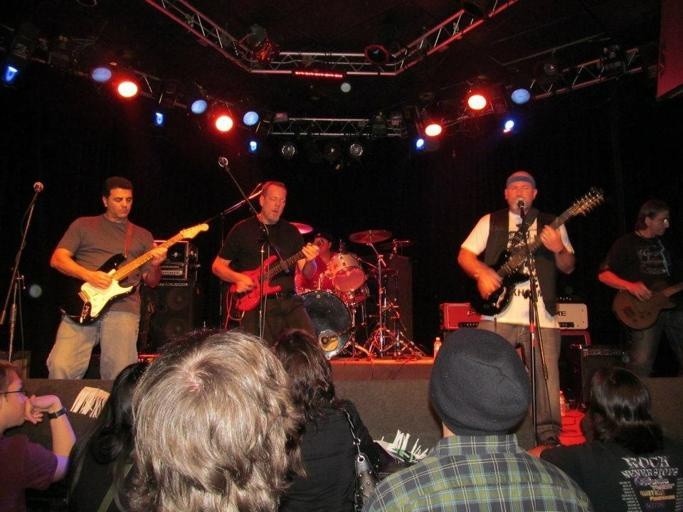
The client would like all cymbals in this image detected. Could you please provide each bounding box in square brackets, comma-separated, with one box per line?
[289, 222, 312, 234]
[379, 239, 409, 248]
[350, 230, 390, 243]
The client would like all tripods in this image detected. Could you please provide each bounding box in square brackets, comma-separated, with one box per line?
[357, 260, 416, 357]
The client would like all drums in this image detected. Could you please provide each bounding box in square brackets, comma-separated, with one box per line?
[329, 254, 365, 292]
[371, 253, 396, 275]
[295, 290, 352, 359]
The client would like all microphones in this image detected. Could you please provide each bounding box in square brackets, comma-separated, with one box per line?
[218, 156, 229, 167]
[517, 200, 525, 218]
[33, 182, 44, 192]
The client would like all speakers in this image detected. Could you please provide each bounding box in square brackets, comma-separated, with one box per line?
[158, 264, 207, 356]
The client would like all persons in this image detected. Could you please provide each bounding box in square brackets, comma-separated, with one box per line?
[46, 174, 168, 380]
[211, 181, 320, 349]
[1, 360, 76, 512]
[539, 367, 682, 512]
[361, 327, 594, 512]
[595, 197, 683, 377]
[313, 231, 338, 272]
[62, 327, 374, 512]
[457, 170, 577, 447]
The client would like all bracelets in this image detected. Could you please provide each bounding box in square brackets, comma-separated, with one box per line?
[555, 246, 567, 255]
[48, 408, 67, 418]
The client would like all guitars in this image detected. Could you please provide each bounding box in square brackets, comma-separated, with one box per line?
[62, 224, 209, 325]
[471, 188, 605, 315]
[613, 282, 682, 329]
[230, 239, 321, 310]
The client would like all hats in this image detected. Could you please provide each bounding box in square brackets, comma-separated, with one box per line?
[428, 327, 530, 437]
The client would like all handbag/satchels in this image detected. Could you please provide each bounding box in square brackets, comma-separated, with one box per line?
[353, 452, 380, 512]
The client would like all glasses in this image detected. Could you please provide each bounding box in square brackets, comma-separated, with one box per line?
[0, 387, 26, 395]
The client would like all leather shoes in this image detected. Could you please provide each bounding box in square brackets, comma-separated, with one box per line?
[535, 437, 560, 447]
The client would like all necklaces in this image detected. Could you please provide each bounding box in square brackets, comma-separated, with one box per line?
[636, 229, 644, 237]
